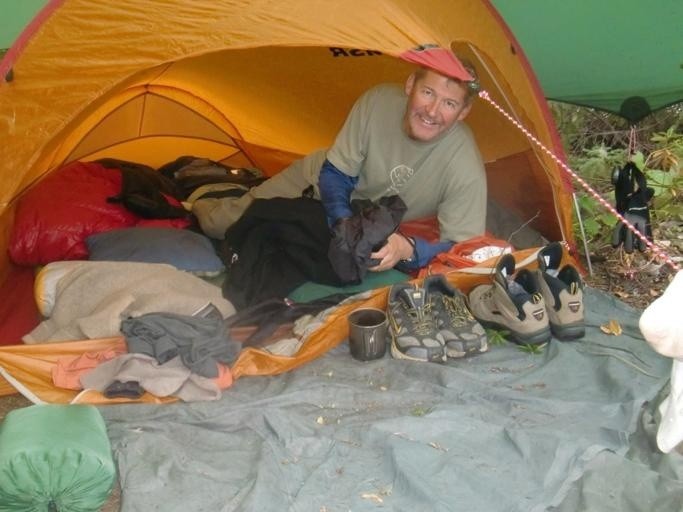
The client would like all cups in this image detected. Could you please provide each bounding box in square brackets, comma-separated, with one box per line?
[347, 307, 388, 361]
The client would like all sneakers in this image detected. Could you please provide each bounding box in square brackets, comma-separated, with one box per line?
[467, 252, 552, 346]
[530, 241, 587, 339]
[385, 281, 449, 363]
[421, 273, 488, 359]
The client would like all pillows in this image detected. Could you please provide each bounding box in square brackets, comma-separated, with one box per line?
[83, 225, 227, 278]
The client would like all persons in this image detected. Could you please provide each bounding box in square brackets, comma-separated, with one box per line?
[187, 58, 488, 273]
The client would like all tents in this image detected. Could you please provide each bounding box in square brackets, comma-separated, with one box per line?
[0, 0, 585, 405]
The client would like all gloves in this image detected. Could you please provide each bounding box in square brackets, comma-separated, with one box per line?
[610, 190, 654, 255]
